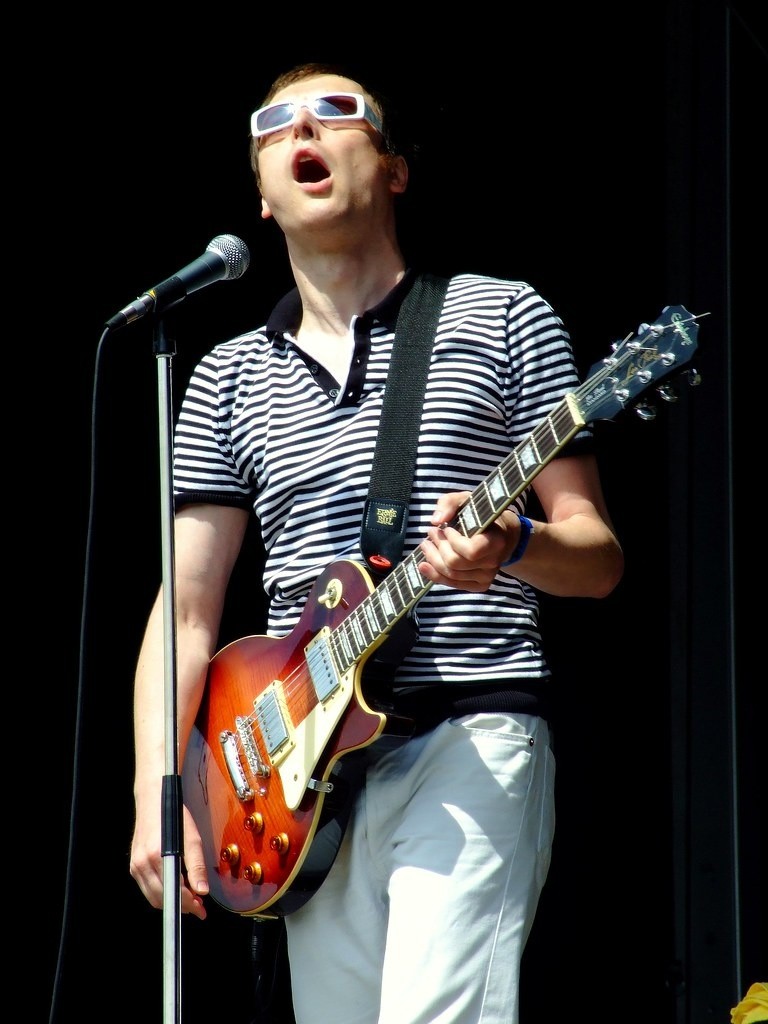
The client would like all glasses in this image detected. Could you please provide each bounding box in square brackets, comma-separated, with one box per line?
[251, 93, 395, 155]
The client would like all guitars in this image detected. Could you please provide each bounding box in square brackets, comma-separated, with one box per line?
[179, 292, 700, 926]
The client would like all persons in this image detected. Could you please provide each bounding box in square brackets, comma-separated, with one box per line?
[129, 64, 626, 1024]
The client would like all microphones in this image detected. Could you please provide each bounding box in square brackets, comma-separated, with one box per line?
[103, 234, 250, 332]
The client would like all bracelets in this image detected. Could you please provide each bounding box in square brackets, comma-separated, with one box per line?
[503, 514, 535, 569]
